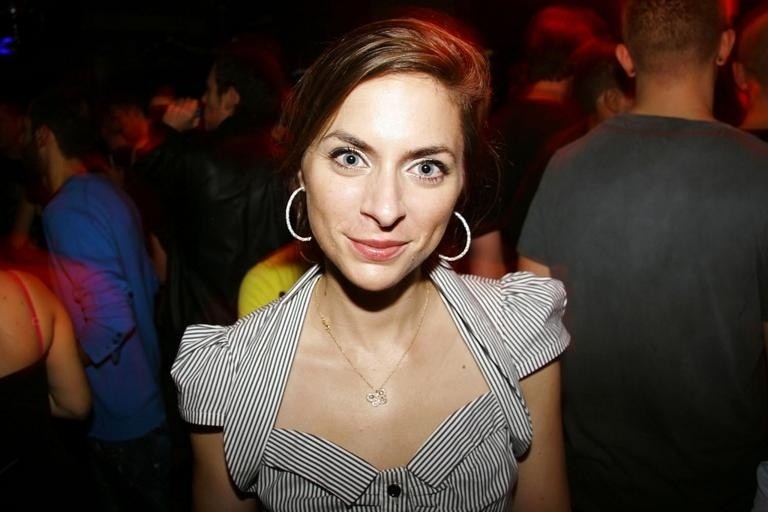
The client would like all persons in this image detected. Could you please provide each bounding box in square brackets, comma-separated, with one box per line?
[0, 1, 768, 512]
